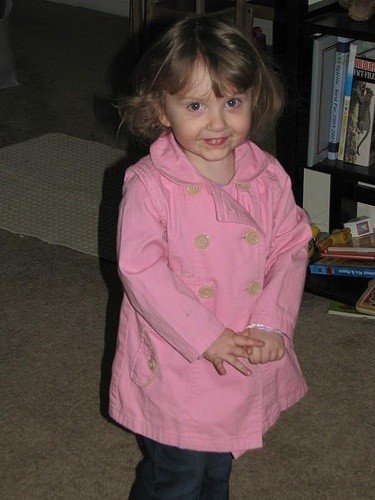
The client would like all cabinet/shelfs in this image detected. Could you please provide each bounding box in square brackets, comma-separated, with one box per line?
[273, 0, 375, 307]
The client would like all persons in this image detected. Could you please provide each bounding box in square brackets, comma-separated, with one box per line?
[108, 15, 312, 500]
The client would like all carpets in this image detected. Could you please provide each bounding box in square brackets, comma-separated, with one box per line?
[0, 132, 143, 264]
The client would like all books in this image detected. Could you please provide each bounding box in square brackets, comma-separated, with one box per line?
[308, 32, 374, 169]
[308, 246, 375, 319]
